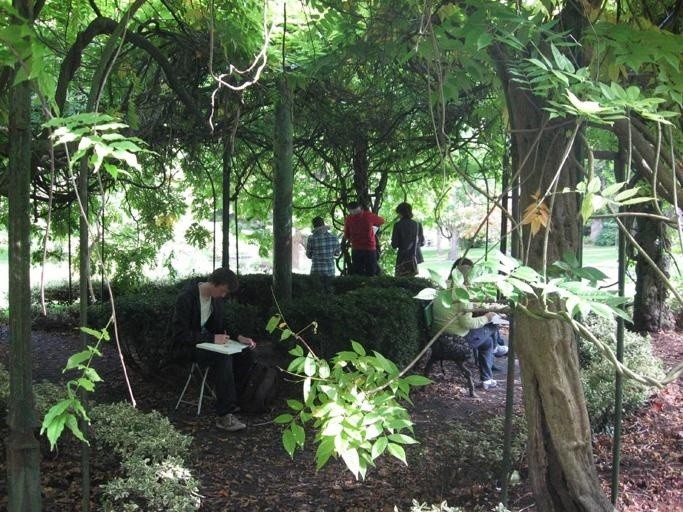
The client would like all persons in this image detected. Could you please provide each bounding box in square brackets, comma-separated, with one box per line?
[304, 216, 339, 277]
[446, 256, 505, 373]
[390, 201, 426, 278]
[343, 201, 384, 276]
[167, 265, 258, 433]
[430, 263, 509, 391]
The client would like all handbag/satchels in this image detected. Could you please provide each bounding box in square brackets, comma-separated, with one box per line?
[396, 256, 418, 280]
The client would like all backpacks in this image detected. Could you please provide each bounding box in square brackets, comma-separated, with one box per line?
[236, 361, 281, 413]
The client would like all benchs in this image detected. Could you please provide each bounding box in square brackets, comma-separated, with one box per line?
[421, 300, 497, 398]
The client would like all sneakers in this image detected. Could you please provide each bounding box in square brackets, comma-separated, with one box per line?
[481, 379, 497, 390]
[494, 346, 509, 357]
[214, 414, 243, 431]
[491, 363, 502, 372]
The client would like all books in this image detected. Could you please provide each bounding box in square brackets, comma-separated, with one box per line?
[195, 338, 250, 356]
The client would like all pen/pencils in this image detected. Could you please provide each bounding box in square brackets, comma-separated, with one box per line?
[224, 330, 226, 339]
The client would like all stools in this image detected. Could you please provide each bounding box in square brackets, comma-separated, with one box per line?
[173, 352, 218, 416]
